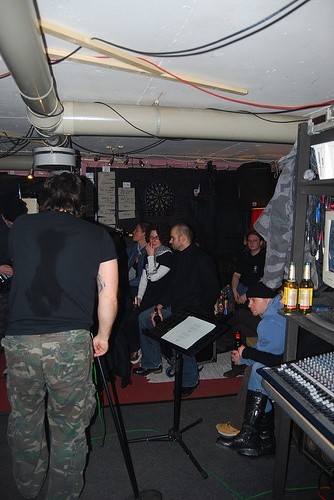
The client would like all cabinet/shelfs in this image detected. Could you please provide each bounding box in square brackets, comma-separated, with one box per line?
[272, 123, 334, 500]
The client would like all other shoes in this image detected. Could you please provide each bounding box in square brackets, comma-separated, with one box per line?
[133, 365, 162, 376]
[223, 369, 244, 378]
[173, 379, 200, 396]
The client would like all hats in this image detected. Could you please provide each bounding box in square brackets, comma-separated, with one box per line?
[2, 198, 27, 222]
[246, 281, 278, 298]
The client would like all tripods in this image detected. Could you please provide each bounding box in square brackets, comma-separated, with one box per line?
[123, 310, 228, 481]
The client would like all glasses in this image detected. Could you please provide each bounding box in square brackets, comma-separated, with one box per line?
[148, 236, 160, 241]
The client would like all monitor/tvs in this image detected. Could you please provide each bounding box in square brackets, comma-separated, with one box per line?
[322, 212, 334, 288]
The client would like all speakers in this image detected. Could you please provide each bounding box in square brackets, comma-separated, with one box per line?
[299, 430, 334, 476]
[237, 162, 272, 202]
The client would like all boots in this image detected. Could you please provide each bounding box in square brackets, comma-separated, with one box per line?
[215, 389, 269, 456]
[238, 399, 276, 458]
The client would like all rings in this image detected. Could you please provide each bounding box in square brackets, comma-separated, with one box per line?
[153, 308, 158, 313]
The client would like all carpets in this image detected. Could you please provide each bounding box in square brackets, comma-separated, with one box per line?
[0, 351, 246, 416]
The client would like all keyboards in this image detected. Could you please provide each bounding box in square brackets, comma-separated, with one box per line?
[306, 309, 334, 331]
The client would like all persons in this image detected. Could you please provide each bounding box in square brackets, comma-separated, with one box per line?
[0, 172, 334, 500]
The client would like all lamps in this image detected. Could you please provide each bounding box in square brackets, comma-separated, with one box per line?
[108, 157, 114, 166]
[139, 159, 145, 167]
[124, 157, 130, 165]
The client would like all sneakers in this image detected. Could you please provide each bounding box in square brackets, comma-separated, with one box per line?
[131, 348, 142, 364]
